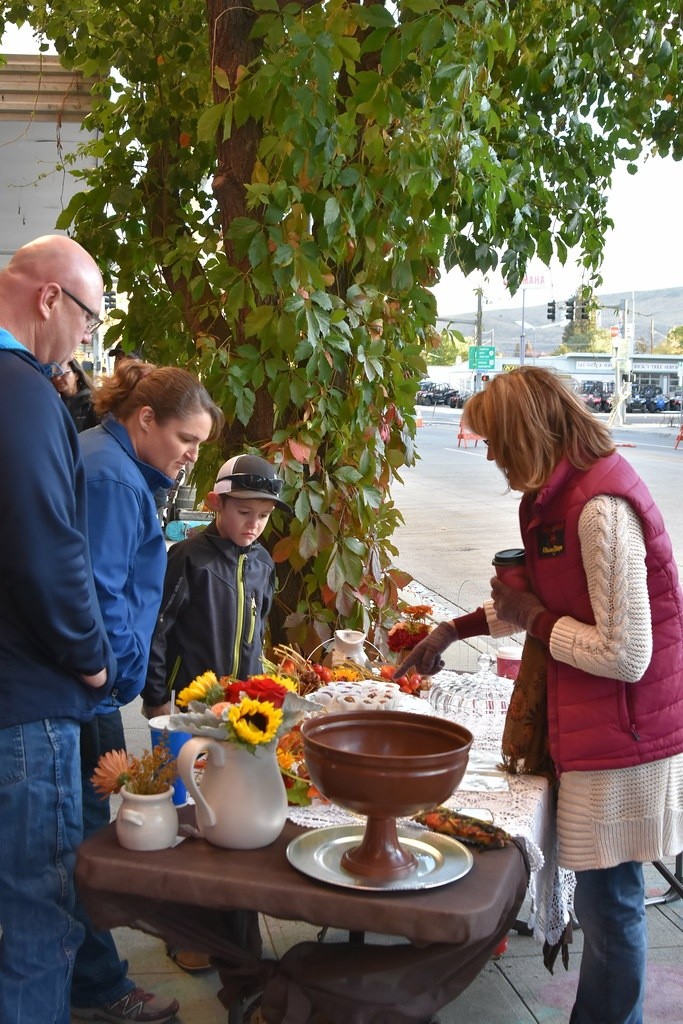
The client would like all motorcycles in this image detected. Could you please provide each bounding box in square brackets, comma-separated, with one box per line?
[416, 382, 471, 408]
[577, 380, 682, 414]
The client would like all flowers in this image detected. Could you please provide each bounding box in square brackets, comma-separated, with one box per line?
[387, 605, 432, 652]
[175, 669, 299, 759]
[90, 725, 207, 802]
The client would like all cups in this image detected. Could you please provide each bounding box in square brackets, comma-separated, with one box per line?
[496, 647, 522, 682]
[149, 716, 192, 809]
[490, 548, 529, 593]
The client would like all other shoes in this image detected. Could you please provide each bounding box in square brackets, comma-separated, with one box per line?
[175, 950, 211, 970]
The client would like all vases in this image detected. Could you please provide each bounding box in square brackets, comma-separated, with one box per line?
[395, 649, 418, 677]
[148, 713, 287, 849]
[322, 628, 371, 668]
[116, 781, 178, 851]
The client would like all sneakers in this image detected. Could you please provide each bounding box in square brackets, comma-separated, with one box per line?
[68, 981, 180, 1024]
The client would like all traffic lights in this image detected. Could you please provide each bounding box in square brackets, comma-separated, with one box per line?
[547, 300, 555, 319]
[482, 376, 489, 381]
[566, 300, 574, 320]
[582, 301, 589, 319]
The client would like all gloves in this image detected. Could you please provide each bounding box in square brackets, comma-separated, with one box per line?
[393, 620, 460, 682]
[489, 576, 545, 636]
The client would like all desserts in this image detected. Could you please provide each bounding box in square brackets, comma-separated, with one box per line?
[314, 681, 400, 710]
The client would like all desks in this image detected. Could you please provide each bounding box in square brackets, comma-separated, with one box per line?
[74, 666, 553, 1024]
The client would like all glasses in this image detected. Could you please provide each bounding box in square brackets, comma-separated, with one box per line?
[38, 284, 104, 335]
[216, 472, 284, 495]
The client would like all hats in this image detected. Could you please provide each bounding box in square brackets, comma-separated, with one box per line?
[214, 454, 294, 514]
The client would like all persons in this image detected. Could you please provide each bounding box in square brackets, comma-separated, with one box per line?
[392, 367, 683, 1024]
[71, 358, 226, 1024]
[0, 235, 117, 1024]
[141, 454, 295, 1024]
[50, 360, 103, 434]
